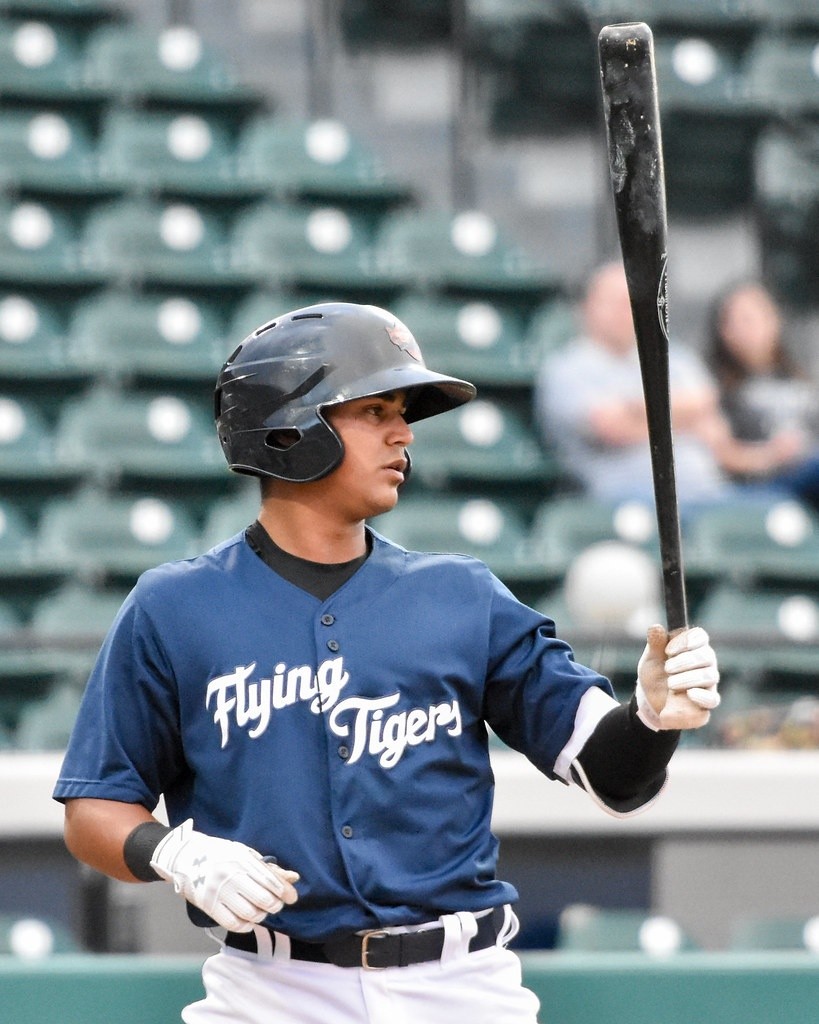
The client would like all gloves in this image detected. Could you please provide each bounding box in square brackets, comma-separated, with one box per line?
[150, 818, 301, 933]
[636, 624, 720, 733]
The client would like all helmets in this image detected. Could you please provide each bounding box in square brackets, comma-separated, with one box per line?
[211, 303, 480, 481]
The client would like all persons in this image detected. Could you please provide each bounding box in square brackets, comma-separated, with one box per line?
[530, 257, 731, 755]
[703, 276, 819, 752]
[48, 300, 722, 1024]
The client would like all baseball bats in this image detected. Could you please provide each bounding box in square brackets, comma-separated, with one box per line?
[591, 18, 691, 696]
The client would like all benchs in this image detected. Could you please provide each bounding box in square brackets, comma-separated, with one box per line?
[0, 0, 819, 709]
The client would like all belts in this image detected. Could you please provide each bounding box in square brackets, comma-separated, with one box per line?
[225, 907, 504, 969]
